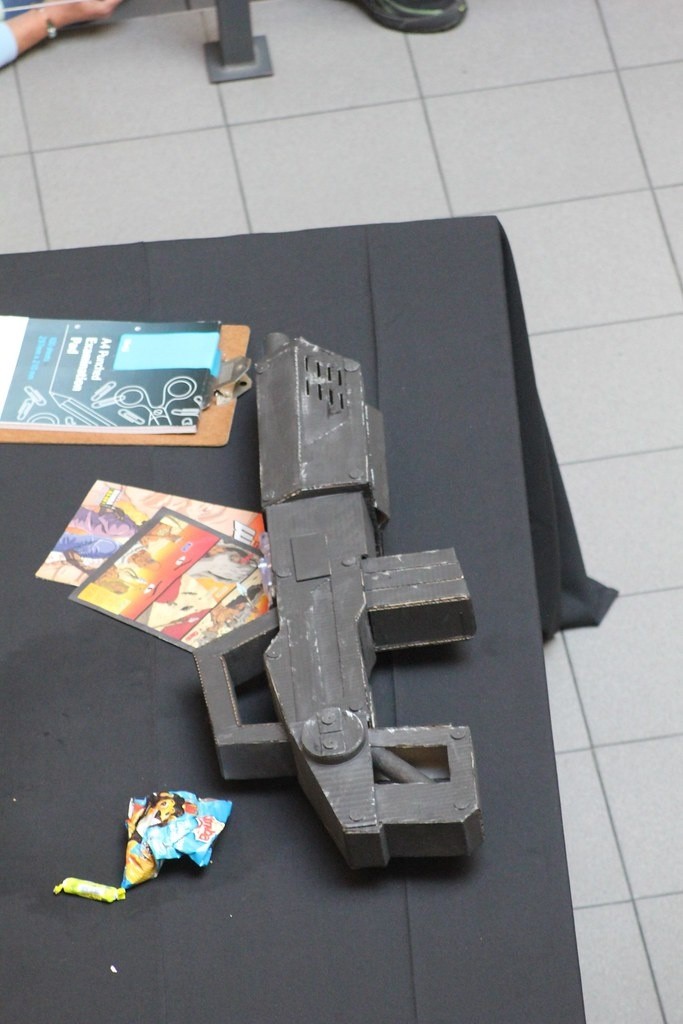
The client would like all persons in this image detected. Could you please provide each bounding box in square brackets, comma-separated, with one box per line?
[0, 0, 122, 69]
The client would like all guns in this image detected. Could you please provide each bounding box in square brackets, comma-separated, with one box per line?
[194, 332, 484, 866]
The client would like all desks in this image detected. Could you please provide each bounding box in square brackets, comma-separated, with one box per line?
[0, 215, 618, 1024]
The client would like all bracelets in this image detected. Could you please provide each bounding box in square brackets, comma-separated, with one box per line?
[39, 8, 56, 39]
